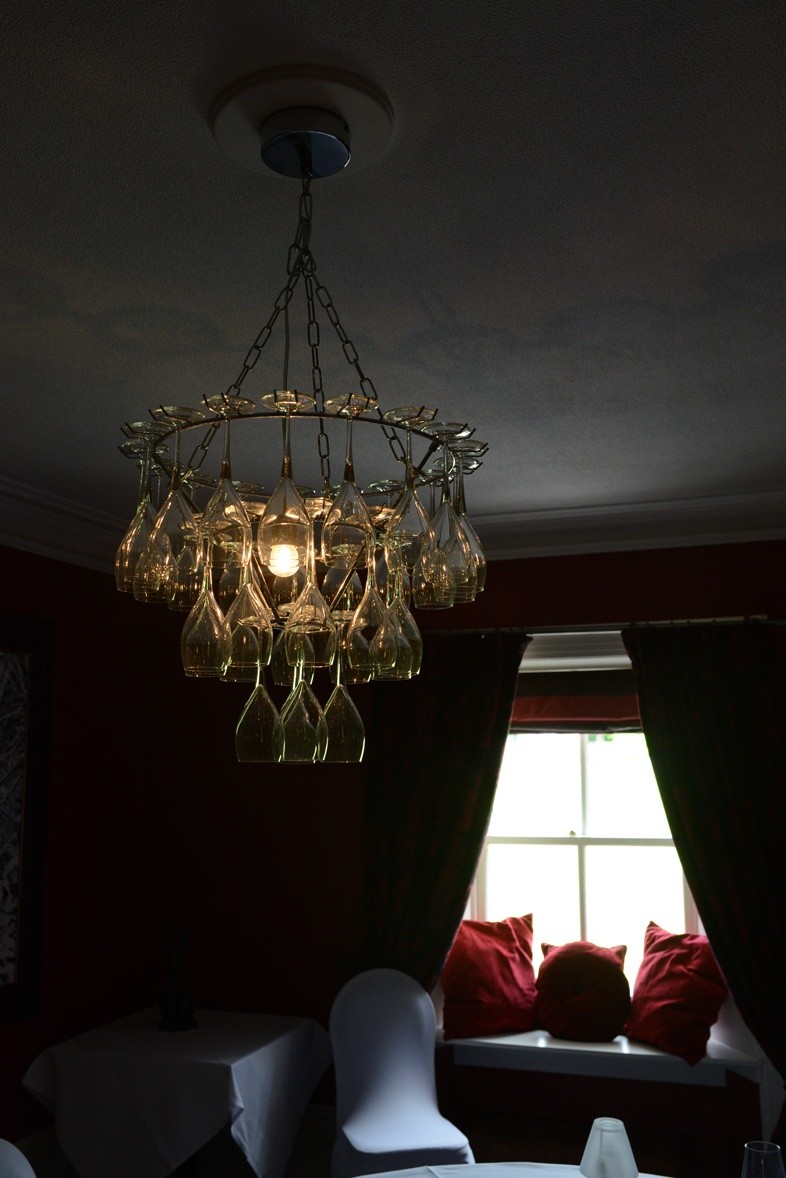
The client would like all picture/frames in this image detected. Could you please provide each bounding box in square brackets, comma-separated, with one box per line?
[0, 613, 55, 1027]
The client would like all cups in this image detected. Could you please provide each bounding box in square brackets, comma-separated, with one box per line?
[579, 1118, 639, 1177]
[741, 1142, 786, 1177]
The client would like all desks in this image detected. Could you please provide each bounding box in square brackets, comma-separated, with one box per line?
[25, 1001, 333, 1178]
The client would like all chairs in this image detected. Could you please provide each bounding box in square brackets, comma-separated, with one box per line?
[325, 968, 475, 1178]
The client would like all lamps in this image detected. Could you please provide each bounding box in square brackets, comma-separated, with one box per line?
[106, 63, 491, 768]
